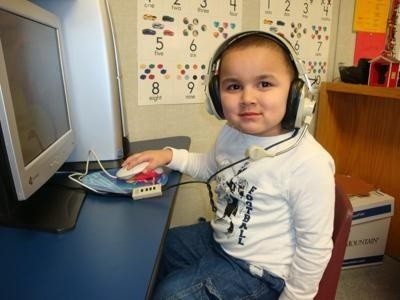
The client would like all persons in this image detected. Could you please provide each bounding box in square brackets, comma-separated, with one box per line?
[120, 29, 337, 300]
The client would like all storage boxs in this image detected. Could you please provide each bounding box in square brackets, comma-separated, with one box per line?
[340, 189, 395, 270]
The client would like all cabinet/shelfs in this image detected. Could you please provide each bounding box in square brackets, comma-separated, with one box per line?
[315, 82, 400, 264]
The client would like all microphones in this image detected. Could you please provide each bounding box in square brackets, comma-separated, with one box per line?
[247, 124, 308, 161]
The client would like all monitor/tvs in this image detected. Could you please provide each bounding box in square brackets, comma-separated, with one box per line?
[0, 0, 87, 234]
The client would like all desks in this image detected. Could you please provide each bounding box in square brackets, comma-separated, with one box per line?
[0, 136, 190, 300]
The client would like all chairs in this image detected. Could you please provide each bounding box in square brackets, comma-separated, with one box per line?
[312, 182, 353, 300]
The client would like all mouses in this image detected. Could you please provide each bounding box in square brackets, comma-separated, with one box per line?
[116, 161, 163, 181]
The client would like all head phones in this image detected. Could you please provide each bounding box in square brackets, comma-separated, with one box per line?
[203, 29, 317, 132]
